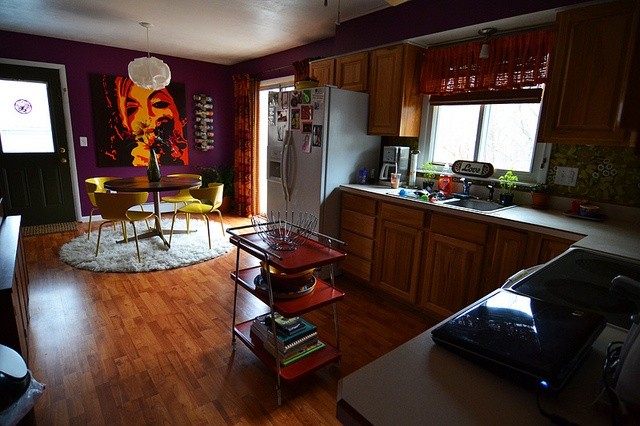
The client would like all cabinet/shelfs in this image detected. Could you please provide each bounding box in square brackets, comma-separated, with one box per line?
[0, 215, 29, 369]
[374, 194, 428, 309]
[536, 0, 640, 148]
[367, 42, 424, 137]
[339, 185, 377, 285]
[309, 48, 369, 92]
[421, 203, 492, 321]
[488, 214, 584, 314]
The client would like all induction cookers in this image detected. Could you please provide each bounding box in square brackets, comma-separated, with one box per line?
[432, 292, 609, 395]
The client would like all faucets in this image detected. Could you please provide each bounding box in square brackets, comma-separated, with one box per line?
[486, 182, 495, 202]
[458, 177, 474, 200]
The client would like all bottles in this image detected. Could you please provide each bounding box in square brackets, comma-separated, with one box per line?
[358, 168, 368, 184]
[439, 161, 453, 195]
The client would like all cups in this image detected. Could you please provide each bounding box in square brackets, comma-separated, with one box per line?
[389, 173, 401, 189]
[358, 169, 366, 184]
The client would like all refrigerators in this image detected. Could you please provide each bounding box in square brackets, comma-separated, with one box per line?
[266, 88, 367, 265]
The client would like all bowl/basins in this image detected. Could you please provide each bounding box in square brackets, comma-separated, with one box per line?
[257, 268, 321, 292]
[579, 204, 600, 217]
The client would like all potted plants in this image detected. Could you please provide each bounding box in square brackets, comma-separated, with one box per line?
[421, 161, 437, 191]
[498, 171, 518, 206]
[529, 183, 549, 209]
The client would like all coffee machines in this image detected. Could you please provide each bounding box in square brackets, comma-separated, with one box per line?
[380, 146, 409, 186]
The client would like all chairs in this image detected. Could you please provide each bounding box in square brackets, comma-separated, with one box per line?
[153, 174, 207, 235]
[169, 182, 225, 249]
[94, 192, 163, 264]
[84, 177, 125, 241]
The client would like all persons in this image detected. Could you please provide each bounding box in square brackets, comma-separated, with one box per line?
[104, 74, 189, 165]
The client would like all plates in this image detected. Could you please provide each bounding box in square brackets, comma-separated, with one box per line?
[251, 278, 318, 302]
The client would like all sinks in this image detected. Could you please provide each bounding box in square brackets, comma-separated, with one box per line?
[446, 193, 502, 214]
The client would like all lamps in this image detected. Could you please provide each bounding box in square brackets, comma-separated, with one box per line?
[478, 27, 497, 59]
[128, 22, 171, 90]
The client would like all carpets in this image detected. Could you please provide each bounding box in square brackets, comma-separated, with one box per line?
[59, 217, 236, 272]
